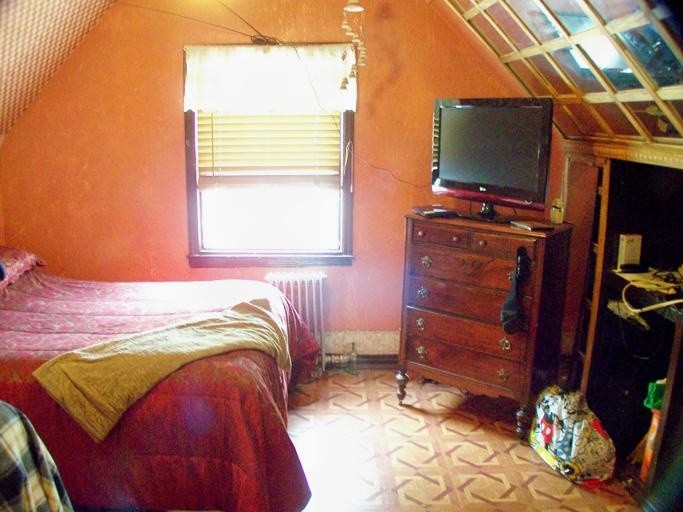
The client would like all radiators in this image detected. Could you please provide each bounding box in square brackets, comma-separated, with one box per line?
[264, 271, 326, 377]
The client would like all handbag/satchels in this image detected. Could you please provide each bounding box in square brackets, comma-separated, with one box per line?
[529, 383, 616, 489]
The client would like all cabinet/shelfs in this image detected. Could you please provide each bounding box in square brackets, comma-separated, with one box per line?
[394, 215, 575, 437]
[549, 139, 682, 503]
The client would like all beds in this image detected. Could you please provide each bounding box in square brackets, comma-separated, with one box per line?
[0, 271, 318, 509]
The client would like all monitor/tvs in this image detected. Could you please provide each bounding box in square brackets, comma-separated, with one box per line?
[432, 97, 554, 223]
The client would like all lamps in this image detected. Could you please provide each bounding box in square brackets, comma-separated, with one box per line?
[339, 0, 366, 12]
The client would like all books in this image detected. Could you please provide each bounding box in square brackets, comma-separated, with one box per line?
[510, 221, 554, 232]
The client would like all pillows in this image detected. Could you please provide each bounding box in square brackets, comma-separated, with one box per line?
[0, 243, 42, 292]
